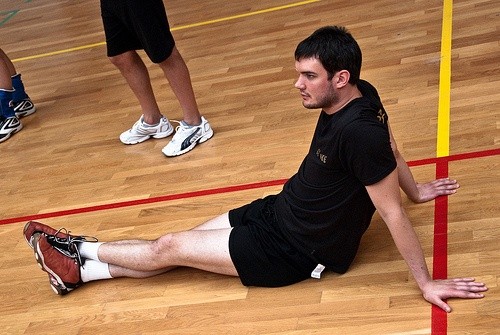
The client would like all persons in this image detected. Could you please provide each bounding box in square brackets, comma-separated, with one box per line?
[100, 0, 213, 156]
[23, 26, 488, 312]
[0, 48, 36, 142]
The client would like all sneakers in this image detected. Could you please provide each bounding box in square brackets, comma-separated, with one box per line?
[118, 115, 174, 143]
[22, 219, 91, 261]
[7, 97, 36, 118]
[27, 234, 83, 296]
[0, 111, 21, 143]
[160, 115, 216, 156]
